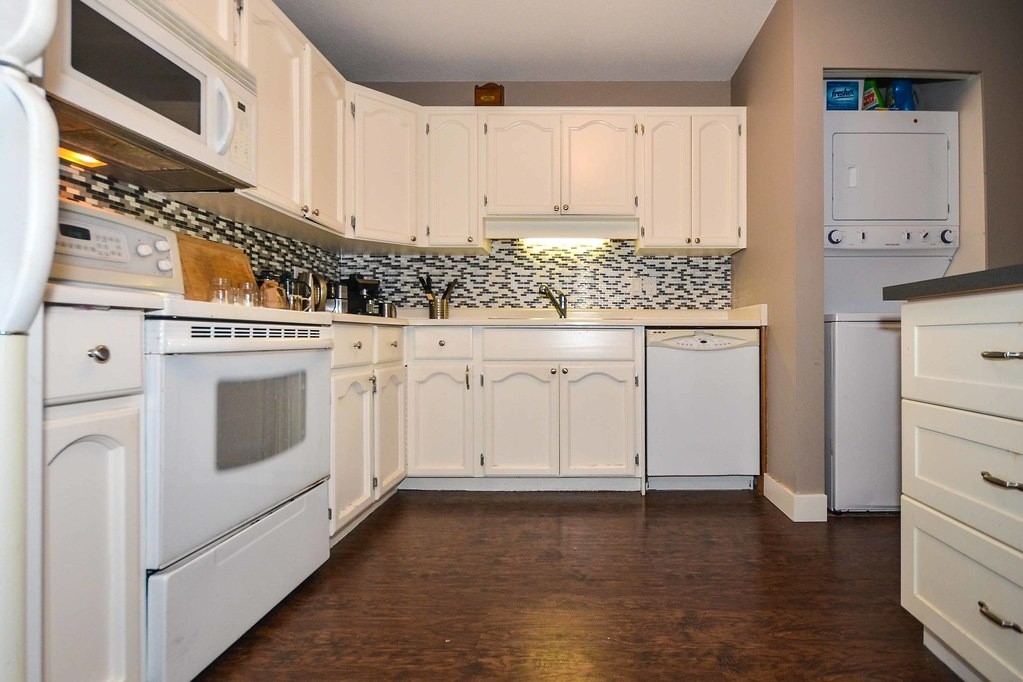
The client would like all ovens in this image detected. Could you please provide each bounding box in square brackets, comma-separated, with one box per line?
[141, 320, 333, 682]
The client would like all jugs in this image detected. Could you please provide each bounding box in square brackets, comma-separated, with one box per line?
[297, 271, 328, 311]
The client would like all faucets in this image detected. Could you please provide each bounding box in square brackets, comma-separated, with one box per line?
[538, 285, 567, 319]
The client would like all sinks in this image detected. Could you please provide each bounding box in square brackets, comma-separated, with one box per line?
[487, 316, 633, 321]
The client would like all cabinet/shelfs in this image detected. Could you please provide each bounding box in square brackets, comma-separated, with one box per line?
[479, 320, 643, 492]
[24, 280, 164, 682]
[45, 0, 749, 255]
[330, 312, 407, 550]
[398, 320, 479, 491]
[882, 264, 1023, 682]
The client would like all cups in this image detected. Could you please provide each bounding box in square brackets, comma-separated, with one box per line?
[378, 302, 397, 318]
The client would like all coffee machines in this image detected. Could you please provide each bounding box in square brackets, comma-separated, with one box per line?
[340, 273, 380, 315]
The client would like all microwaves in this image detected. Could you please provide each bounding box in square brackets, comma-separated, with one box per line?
[41, 1, 259, 188]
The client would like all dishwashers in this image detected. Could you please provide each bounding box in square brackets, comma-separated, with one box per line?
[647, 329, 760, 490]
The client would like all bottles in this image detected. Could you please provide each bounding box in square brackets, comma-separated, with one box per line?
[206, 270, 311, 311]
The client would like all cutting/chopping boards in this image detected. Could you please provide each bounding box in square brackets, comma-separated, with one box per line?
[175, 232, 262, 302]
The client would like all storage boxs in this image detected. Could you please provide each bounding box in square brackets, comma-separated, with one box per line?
[475, 82, 504, 106]
[823, 79, 865, 111]
